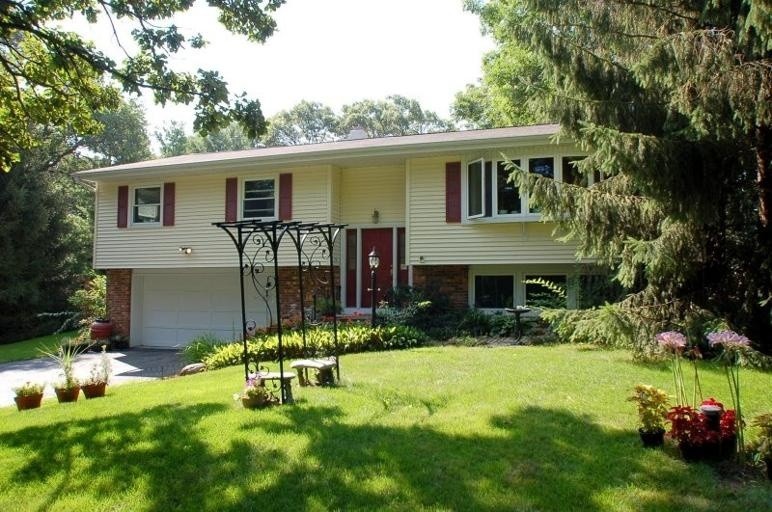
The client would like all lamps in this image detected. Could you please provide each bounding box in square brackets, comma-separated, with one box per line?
[371, 208, 380, 224]
[176, 247, 192, 255]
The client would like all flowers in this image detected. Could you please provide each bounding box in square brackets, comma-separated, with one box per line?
[625, 382, 672, 433]
[698, 397, 737, 443]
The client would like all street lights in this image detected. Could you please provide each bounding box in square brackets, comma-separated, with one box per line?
[366, 245, 382, 329]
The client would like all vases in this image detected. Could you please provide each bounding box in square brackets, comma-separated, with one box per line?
[638, 427, 665, 447]
[701, 436, 738, 467]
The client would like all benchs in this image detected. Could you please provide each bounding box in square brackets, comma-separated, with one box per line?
[290, 359, 337, 387]
[245, 372, 296, 404]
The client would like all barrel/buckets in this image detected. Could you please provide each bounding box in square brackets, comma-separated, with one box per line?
[91, 318, 112, 352]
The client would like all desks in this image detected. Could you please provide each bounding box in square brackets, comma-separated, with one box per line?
[505, 308, 531, 340]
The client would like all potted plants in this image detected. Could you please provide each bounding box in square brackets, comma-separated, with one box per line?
[81, 345, 111, 399]
[669, 406, 702, 459]
[241, 387, 266, 408]
[34, 334, 97, 403]
[11, 382, 46, 411]
